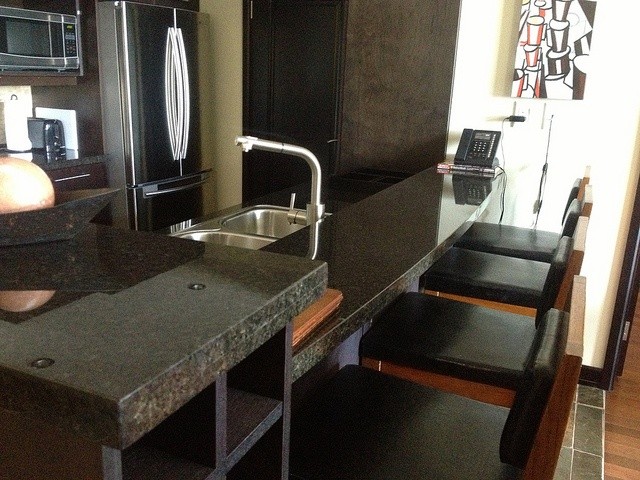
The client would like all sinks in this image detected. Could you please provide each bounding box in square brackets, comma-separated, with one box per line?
[170, 231, 279, 251]
[216, 203, 332, 239]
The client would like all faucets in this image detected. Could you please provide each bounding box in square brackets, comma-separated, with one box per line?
[234, 135, 325, 226]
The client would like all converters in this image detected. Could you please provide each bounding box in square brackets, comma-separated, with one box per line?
[509, 116, 526, 122]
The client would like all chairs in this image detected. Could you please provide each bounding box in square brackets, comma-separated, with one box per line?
[378, 216, 593, 400]
[358, 274, 588, 479]
[459, 167, 595, 263]
[421, 216, 591, 315]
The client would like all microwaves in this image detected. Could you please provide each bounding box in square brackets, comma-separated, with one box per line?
[0, 5, 80, 71]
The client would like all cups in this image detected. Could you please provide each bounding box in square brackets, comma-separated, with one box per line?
[545, 20, 570, 52]
[511, 68, 530, 99]
[522, 44, 543, 67]
[546, 46, 571, 75]
[552, 0, 573, 23]
[563, 54, 591, 100]
[539, 6, 553, 31]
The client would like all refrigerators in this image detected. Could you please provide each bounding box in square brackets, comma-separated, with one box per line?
[96, 0, 218, 232]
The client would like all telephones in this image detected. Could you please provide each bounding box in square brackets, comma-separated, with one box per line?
[453, 128, 502, 168]
[452, 174, 493, 205]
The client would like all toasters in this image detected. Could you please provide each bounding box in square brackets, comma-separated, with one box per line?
[30, 149, 66, 167]
[28, 117, 67, 152]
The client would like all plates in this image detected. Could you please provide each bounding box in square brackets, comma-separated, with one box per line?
[0, 187, 124, 246]
